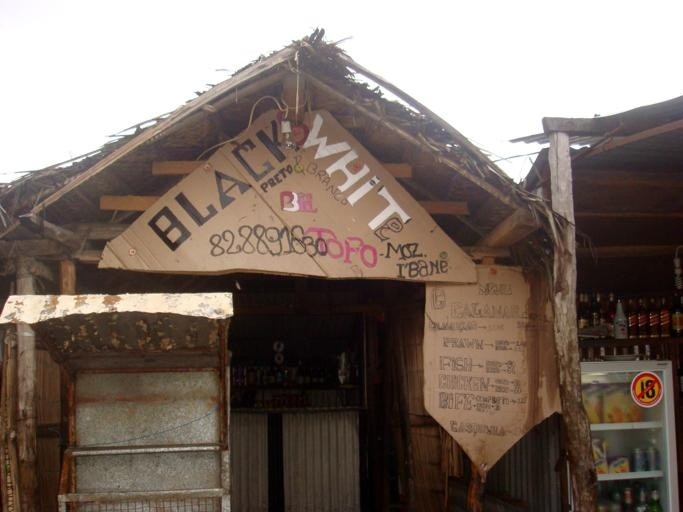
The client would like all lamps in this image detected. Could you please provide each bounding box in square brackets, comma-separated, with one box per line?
[279, 120, 296, 152]
[671, 242, 682, 292]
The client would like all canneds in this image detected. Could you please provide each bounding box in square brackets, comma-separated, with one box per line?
[631, 446, 659, 472]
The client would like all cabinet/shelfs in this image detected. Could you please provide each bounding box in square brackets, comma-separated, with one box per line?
[560, 361, 681, 512]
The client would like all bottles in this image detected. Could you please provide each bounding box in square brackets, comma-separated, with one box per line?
[579, 289, 683, 362]
[597, 480, 664, 512]
